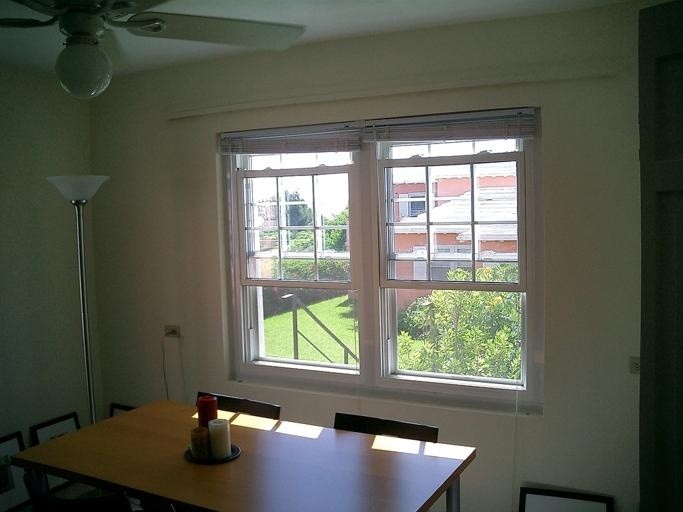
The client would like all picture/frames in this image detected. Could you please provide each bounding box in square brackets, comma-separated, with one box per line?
[29, 411, 81, 494]
[0, 430, 30, 512]
[519, 486, 614, 512]
[109, 403, 136, 417]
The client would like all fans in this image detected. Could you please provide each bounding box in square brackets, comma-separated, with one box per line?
[0, 0, 310, 101]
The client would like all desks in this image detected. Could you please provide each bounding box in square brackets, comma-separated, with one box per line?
[9, 398, 478, 512]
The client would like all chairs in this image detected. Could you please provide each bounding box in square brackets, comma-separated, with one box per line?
[333, 413, 439, 443]
[195, 392, 281, 420]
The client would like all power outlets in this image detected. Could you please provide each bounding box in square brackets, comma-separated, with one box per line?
[630, 356, 641, 373]
[165, 324, 181, 338]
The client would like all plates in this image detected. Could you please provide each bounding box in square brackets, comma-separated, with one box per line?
[185, 444, 242, 466]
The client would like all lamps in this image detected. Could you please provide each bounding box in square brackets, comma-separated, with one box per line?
[46, 173, 111, 427]
[54, 43, 117, 100]
